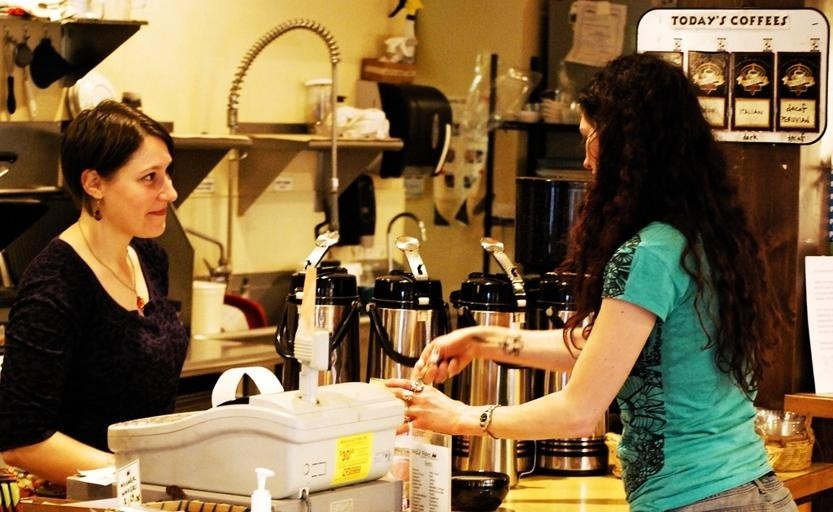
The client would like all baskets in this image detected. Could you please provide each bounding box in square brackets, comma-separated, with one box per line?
[761, 430, 814, 471]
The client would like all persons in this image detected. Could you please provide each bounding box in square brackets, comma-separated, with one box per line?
[1, 102, 187, 500]
[385, 52, 799, 510]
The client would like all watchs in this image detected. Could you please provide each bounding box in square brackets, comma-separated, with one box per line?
[477, 402, 504, 442]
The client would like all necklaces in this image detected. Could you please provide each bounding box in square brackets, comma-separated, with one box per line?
[76, 218, 145, 311]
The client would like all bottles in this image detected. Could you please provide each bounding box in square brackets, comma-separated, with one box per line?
[366, 232, 449, 397]
[267, 229, 364, 395]
[519, 263, 611, 478]
[451, 233, 539, 487]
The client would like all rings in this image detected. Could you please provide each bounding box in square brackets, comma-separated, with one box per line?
[410, 378, 427, 393]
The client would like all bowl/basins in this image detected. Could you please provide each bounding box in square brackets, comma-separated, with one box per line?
[448, 469, 512, 512]
